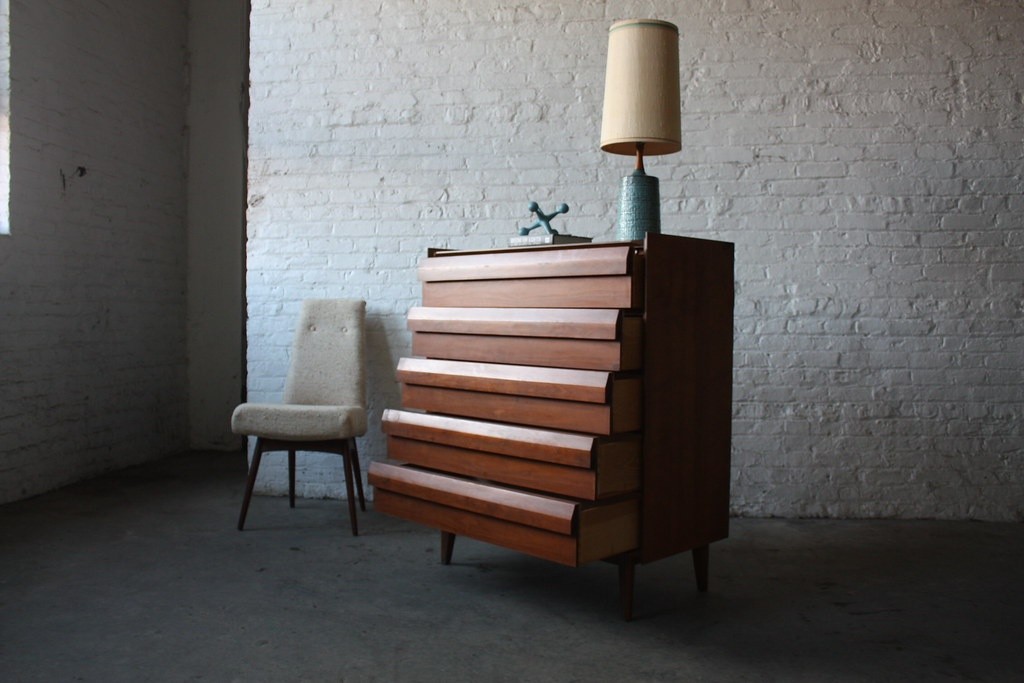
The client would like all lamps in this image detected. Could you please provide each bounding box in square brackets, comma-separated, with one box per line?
[600, 19, 687, 243]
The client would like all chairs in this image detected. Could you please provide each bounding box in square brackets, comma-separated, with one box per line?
[230, 293, 369, 543]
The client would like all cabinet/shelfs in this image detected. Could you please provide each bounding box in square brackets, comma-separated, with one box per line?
[370, 234, 736, 624]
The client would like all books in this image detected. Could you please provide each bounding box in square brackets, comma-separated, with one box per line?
[506, 234, 592, 246]
